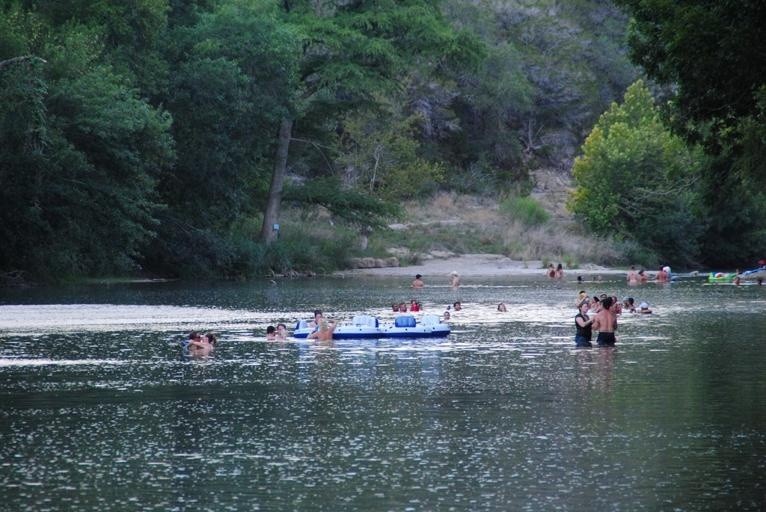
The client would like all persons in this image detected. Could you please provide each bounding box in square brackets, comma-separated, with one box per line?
[734, 264, 763, 285]
[183, 332, 217, 353]
[412, 274, 424, 287]
[546, 263, 671, 347]
[498, 303, 506, 312]
[450, 271, 459, 287]
[267, 298, 460, 342]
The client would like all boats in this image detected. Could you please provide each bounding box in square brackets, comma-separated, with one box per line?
[292, 309, 450, 339]
